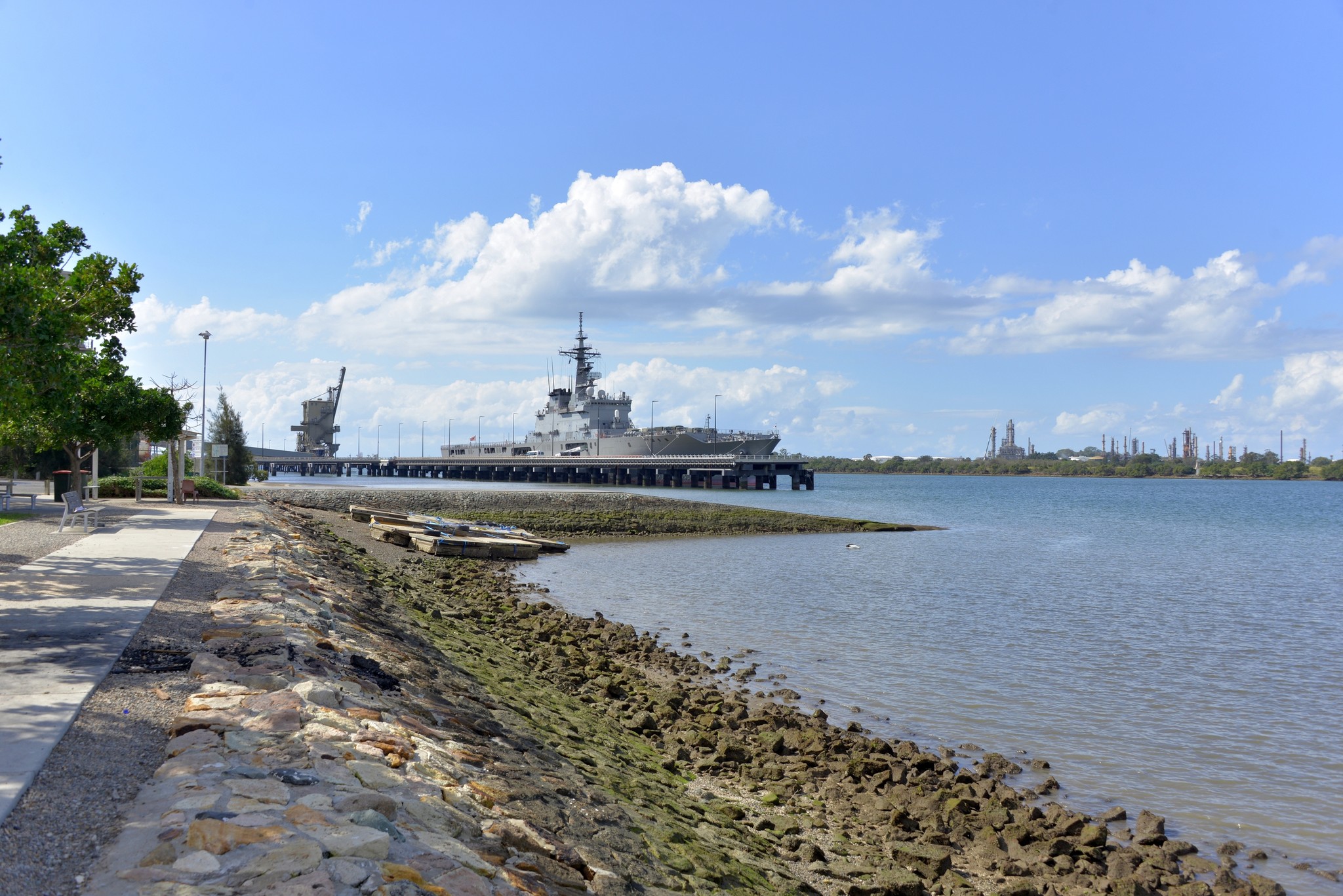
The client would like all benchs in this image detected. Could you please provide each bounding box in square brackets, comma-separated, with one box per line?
[58, 490, 106, 533]
[0, 495, 12, 511]
[83, 485, 101, 501]
[0, 492, 42, 510]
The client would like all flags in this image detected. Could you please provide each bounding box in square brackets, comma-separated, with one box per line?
[469, 435, 476, 441]
[773, 425, 776, 428]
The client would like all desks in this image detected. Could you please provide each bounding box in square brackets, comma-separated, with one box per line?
[0, 480, 31, 511]
[133, 476, 175, 501]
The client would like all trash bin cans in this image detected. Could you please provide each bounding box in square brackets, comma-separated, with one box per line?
[52, 470, 71, 502]
[80, 470, 91, 500]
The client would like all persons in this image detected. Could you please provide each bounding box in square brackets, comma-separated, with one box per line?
[505, 439, 507, 444]
[525, 435, 528, 443]
[470, 442, 471, 446]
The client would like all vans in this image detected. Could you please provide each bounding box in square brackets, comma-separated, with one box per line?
[526, 450, 544, 456]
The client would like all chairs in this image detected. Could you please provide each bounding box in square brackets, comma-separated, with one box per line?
[180, 479, 199, 504]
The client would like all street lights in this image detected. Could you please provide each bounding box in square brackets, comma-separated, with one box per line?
[449, 419, 455, 457]
[262, 423, 265, 458]
[651, 401, 659, 455]
[257, 441, 259, 448]
[422, 421, 427, 457]
[197, 330, 214, 478]
[358, 427, 363, 457]
[714, 394, 723, 456]
[284, 439, 286, 450]
[552, 410, 559, 456]
[597, 405, 605, 455]
[479, 416, 485, 456]
[269, 440, 271, 449]
[377, 425, 383, 455]
[512, 413, 519, 456]
[399, 423, 404, 457]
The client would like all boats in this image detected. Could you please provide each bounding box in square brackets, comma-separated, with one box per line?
[440, 312, 782, 459]
